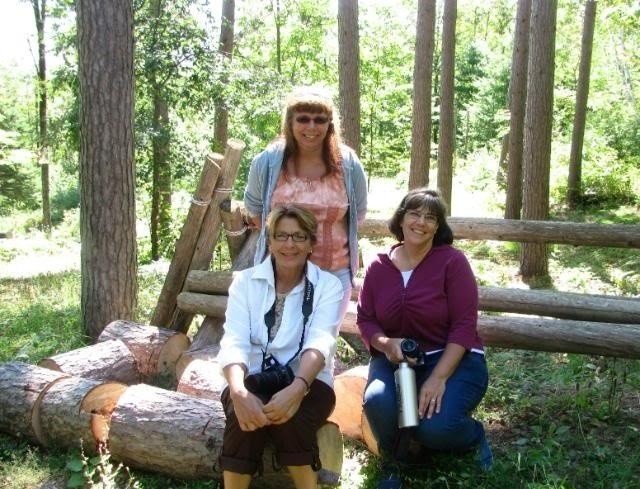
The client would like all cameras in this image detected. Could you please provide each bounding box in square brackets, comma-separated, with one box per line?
[245, 354, 295, 404]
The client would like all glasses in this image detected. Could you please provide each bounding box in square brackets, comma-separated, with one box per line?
[273, 232, 309, 242]
[406, 210, 438, 224]
[295, 115, 328, 124]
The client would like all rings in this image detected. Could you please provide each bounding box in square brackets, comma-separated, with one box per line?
[432, 397, 438, 403]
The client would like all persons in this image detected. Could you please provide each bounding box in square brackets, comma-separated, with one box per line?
[220, 203, 347, 488]
[245, 86, 369, 342]
[356, 186, 494, 488]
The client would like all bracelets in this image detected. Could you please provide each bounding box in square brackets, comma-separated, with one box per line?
[296, 376, 311, 396]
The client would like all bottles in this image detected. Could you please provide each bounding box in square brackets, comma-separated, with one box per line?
[393, 361, 420, 430]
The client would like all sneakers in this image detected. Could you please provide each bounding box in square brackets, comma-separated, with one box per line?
[473, 422, 495, 473]
